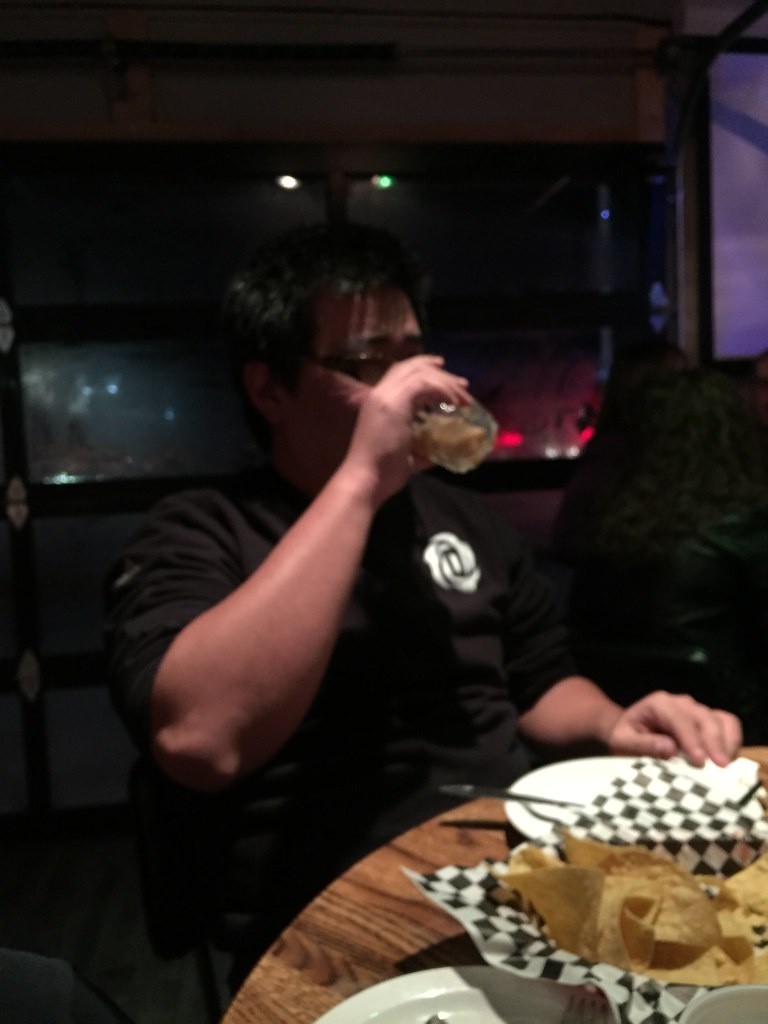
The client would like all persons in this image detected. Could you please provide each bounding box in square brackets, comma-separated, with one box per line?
[98, 228, 744, 1001]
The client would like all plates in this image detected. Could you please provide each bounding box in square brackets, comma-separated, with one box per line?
[502, 754, 768, 848]
[313, 963, 622, 1024]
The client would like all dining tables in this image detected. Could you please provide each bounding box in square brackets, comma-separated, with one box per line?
[224, 742, 768, 1024]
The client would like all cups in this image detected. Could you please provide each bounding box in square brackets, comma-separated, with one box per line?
[678, 983, 768, 1024]
[409, 393, 497, 474]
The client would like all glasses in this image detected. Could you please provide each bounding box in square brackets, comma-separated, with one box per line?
[311, 348, 399, 386]
